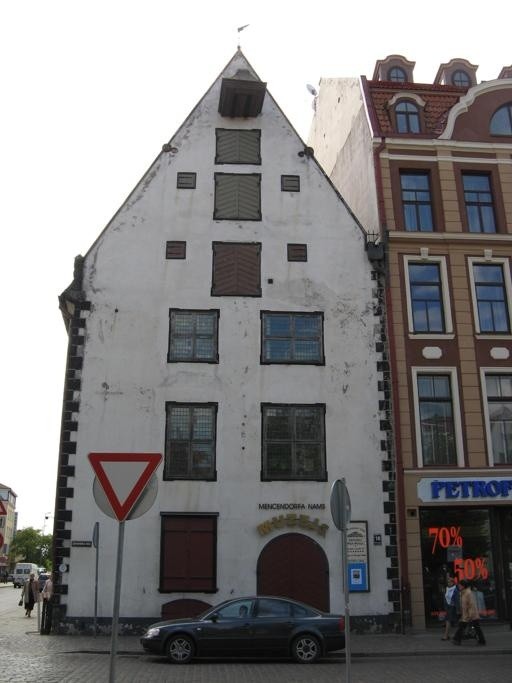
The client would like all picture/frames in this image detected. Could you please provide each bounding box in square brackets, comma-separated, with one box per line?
[345, 520, 370, 592]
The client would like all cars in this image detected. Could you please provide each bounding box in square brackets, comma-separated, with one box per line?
[12, 561, 50, 589]
[140, 592, 348, 665]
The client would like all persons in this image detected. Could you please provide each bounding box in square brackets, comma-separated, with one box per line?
[463, 583, 486, 640]
[238, 605, 248, 618]
[439, 577, 459, 640]
[21, 572, 41, 618]
[42, 572, 54, 612]
[4, 568, 9, 584]
[452, 579, 487, 647]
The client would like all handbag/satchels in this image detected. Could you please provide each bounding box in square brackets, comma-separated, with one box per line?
[18, 596, 24, 606]
[460, 623, 478, 640]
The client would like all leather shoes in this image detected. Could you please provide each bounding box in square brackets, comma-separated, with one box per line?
[442, 636, 485, 645]
